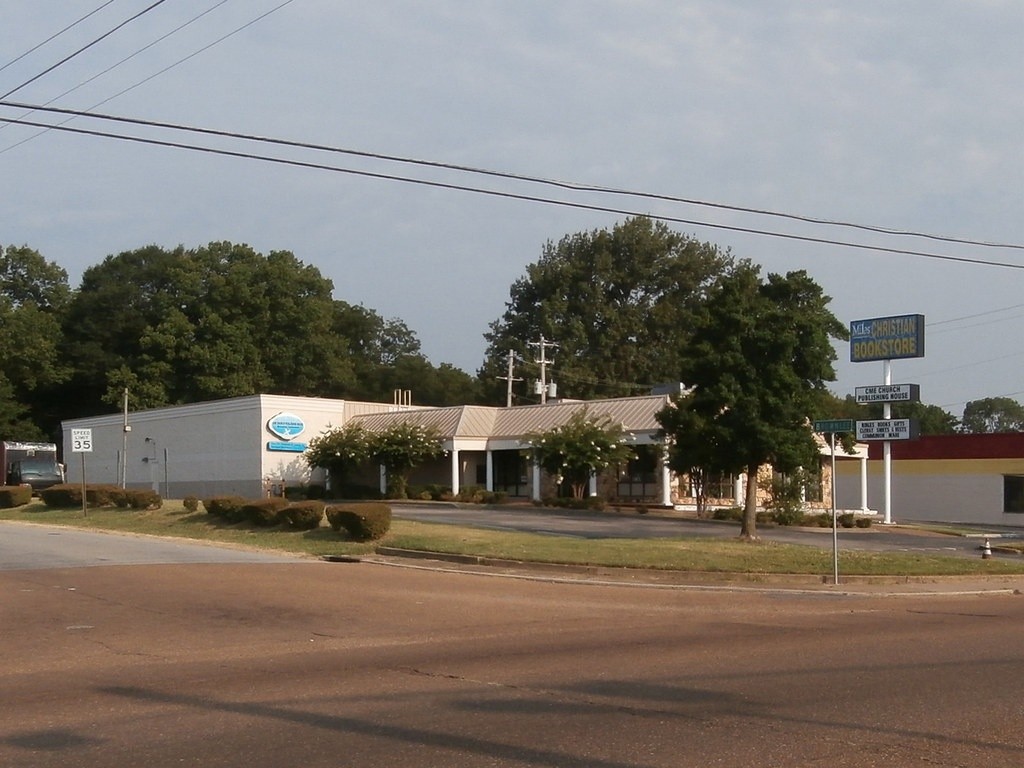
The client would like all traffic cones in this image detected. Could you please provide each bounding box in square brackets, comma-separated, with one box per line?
[980, 538, 992, 560]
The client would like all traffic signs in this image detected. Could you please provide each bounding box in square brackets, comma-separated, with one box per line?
[815, 419, 852, 432]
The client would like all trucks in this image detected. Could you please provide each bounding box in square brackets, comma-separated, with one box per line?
[0, 440, 66, 499]
